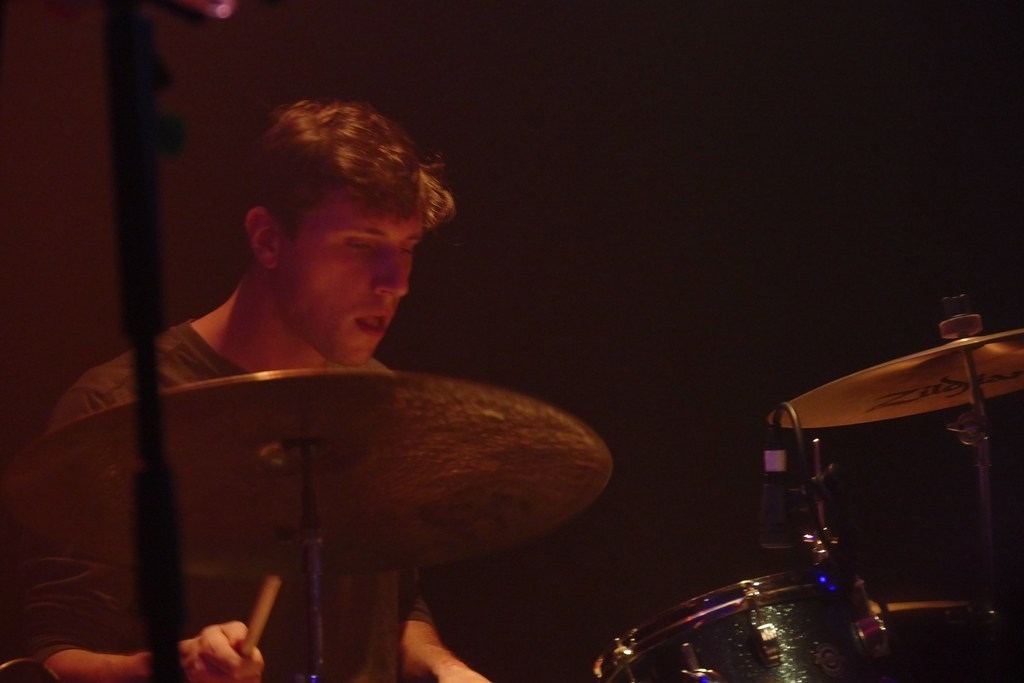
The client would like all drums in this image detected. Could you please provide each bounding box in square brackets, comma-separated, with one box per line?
[592, 569, 888, 683]
[855, 602, 1005, 683]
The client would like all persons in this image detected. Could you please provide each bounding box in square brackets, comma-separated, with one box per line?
[37, 100, 492, 683]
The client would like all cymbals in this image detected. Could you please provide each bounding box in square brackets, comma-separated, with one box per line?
[769, 314, 1024, 431]
[1, 364, 615, 578]
